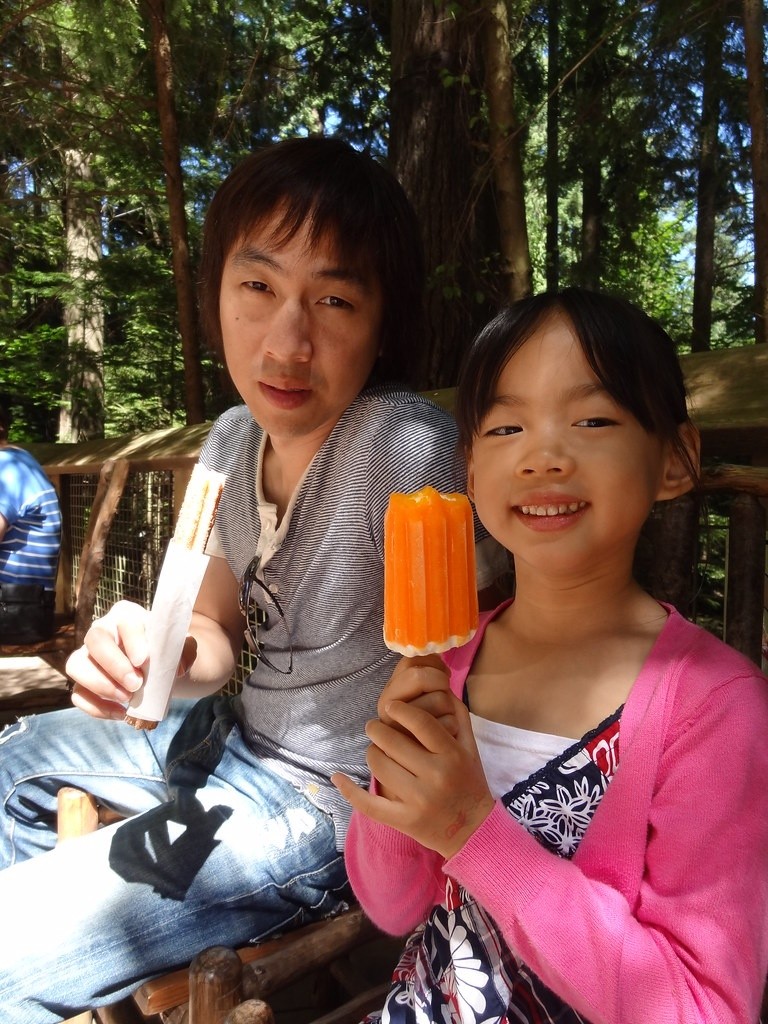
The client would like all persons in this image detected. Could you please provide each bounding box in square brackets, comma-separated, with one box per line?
[0, 133, 516, 1024]
[330, 282, 768, 1024]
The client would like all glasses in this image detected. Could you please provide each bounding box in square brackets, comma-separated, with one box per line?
[238, 554, 295, 675]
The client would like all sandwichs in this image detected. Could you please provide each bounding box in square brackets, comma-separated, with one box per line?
[124, 463, 227, 730]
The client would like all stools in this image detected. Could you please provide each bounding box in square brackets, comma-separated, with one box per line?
[1, 613, 76, 657]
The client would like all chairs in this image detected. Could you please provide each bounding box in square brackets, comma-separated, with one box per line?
[56, 466, 768, 1024]
[1, 456, 130, 732]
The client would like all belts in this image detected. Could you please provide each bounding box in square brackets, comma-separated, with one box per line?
[0, 584, 57, 603]
